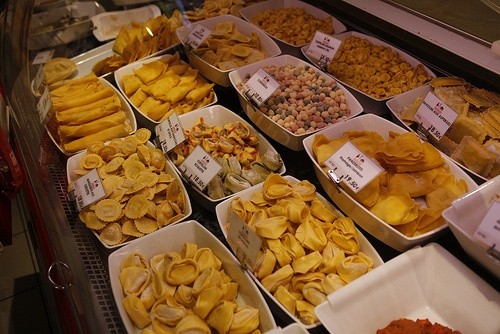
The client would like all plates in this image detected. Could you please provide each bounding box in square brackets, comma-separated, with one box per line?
[301, 31, 437, 101]
[91, 5, 161, 42]
[238, 0, 347, 47]
[314, 242, 500, 334]
[441, 174, 500, 277]
[67, 134, 192, 249]
[302, 113, 478, 252]
[176, 14, 282, 87]
[114, 54, 218, 124]
[228, 54, 364, 151]
[44, 77, 137, 156]
[108, 220, 277, 334]
[277, 323, 311, 334]
[215, 175, 385, 329]
[155, 104, 286, 202]
[386, 76, 500, 181]
[31, 39, 181, 97]
